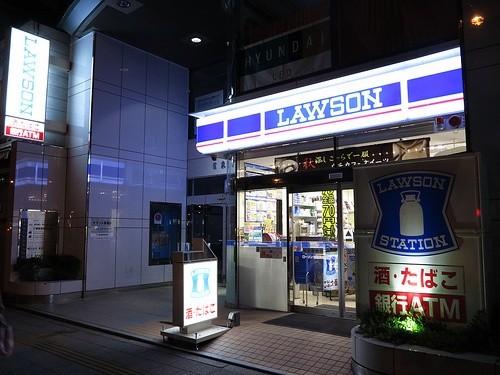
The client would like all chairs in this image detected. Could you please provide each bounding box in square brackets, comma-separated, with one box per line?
[294, 251, 318, 305]
